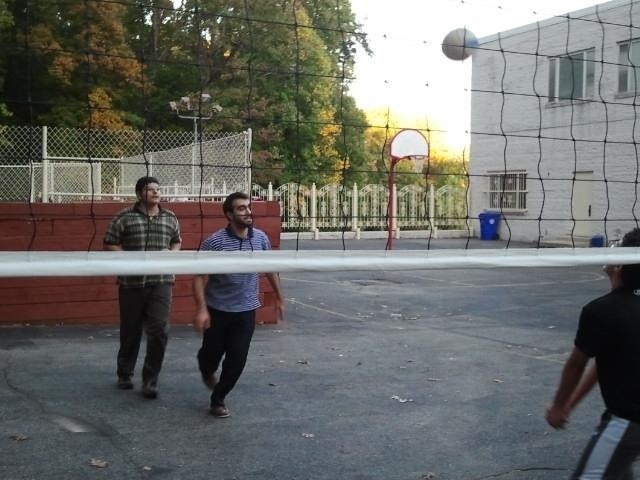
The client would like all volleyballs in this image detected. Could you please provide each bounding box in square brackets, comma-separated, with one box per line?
[443, 28, 478, 60]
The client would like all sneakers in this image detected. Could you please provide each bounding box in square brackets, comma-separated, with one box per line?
[211, 402, 230, 418]
[202, 371, 220, 390]
[142, 381, 159, 397]
[118, 375, 133, 390]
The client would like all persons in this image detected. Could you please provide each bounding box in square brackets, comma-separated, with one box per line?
[103, 177, 182, 398]
[191, 188, 286, 416]
[542, 228, 640, 479]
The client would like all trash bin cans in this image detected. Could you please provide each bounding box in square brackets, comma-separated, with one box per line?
[478, 212, 502, 241]
